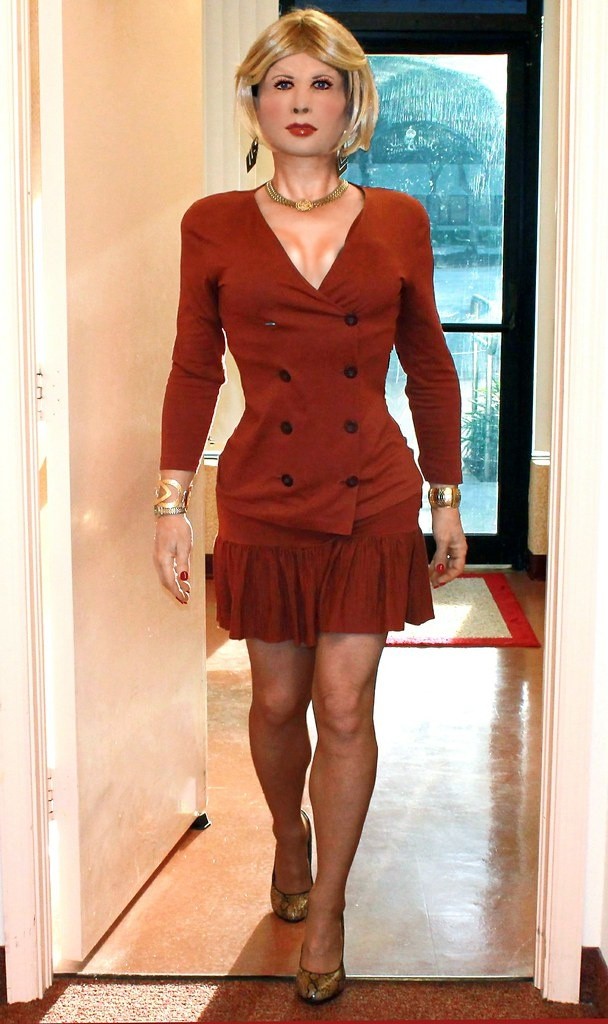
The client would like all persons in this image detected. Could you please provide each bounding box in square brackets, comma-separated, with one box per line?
[152, 9, 468, 1003]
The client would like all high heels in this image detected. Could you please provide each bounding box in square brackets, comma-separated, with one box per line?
[271, 811, 314, 923]
[297, 911, 345, 1004]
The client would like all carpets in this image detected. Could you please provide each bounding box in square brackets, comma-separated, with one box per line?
[385, 568, 544, 650]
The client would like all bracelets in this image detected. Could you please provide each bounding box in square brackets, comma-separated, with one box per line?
[153, 507, 186, 516]
[428, 487, 462, 509]
[154, 475, 193, 508]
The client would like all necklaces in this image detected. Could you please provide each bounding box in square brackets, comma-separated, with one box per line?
[265, 180, 349, 211]
